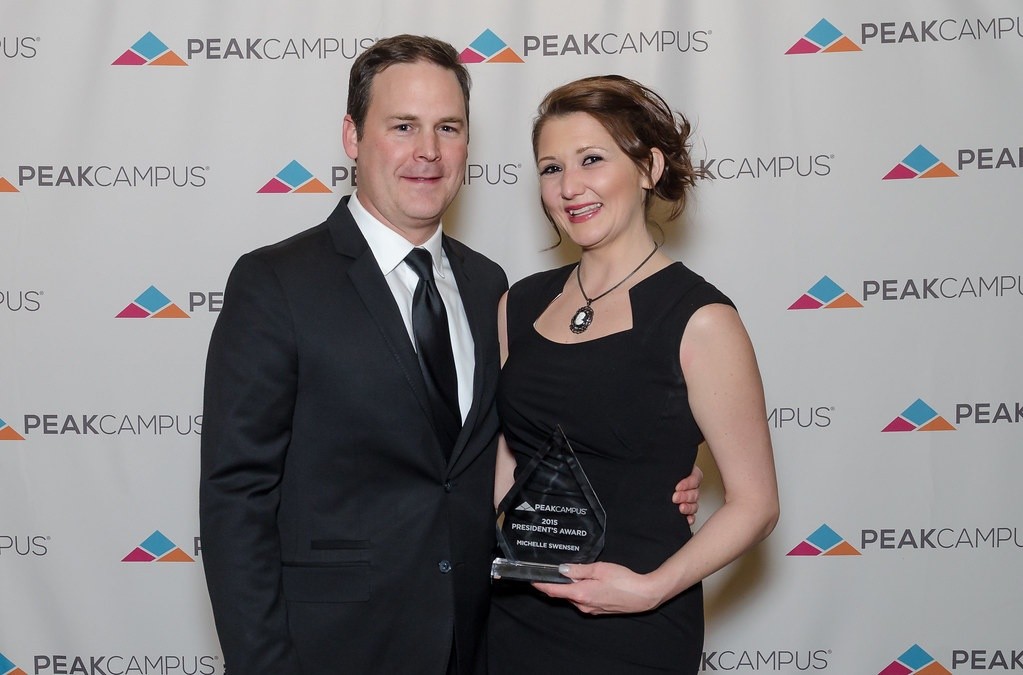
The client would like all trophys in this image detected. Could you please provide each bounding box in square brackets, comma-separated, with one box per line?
[490, 423, 609, 584]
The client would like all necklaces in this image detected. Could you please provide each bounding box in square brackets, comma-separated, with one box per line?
[569, 242, 657, 334]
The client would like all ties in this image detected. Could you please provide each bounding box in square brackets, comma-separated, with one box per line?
[402, 247, 463, 450]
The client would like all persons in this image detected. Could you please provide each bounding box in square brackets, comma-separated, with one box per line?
[195, 32, 701, 675]
[494, 76, 778, 674]
